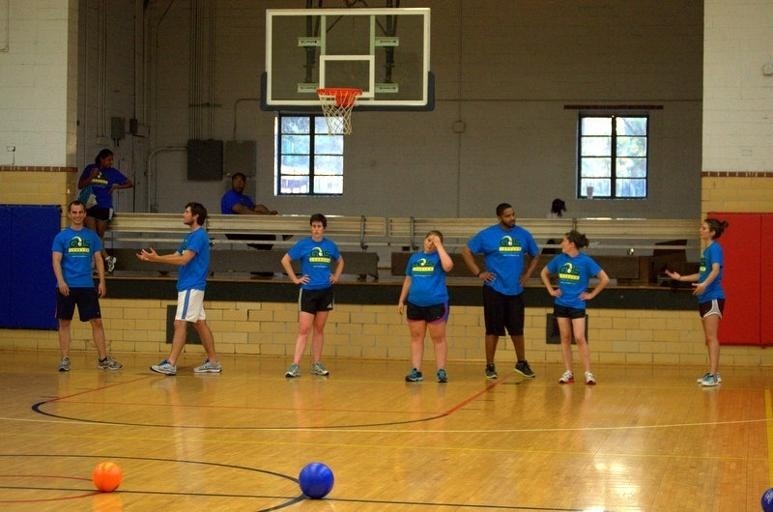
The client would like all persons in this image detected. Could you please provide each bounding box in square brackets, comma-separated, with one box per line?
[462, 203, 540, 378]
[540, 230, 610, 385]
[280, 213, 345, 377]
[220, 171, 300, 279]
[78, 149, 133, 275]
[665, 218, 729, 386]
[398, 229, 454, 383]
[136, 202, 222, 375]
[51, 200, 123, 372]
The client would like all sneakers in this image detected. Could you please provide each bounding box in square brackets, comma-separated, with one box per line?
[96, 356, 123, 371]
[512, 360, 536, 378]
[308, 363, 330, 376]
[701, 374, 718, 386]
[105, 255, 117, 273]
[583, 372, 596, 384]
[58, 356, 73, 372]
[483, 363, 498, 379]
[558, 370, 575, 384]
[284, 363, 301, 378]
[436, 368, 448, 384]
[193, 358, 222, 373]
[405, 368, 422, 382]
[697, 372, 722, 383]
[149, 361, 178, 377]
